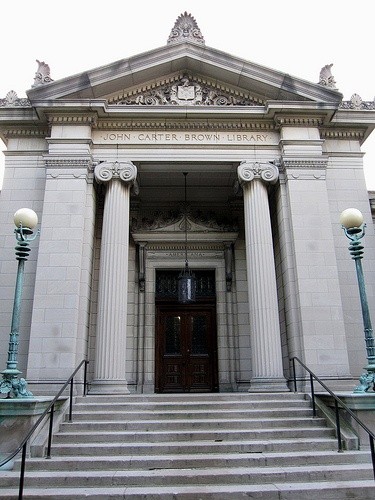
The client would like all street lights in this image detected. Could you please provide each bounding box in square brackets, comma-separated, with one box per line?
[340, 208, 375, 392]
[1, 208, 40, 398]
